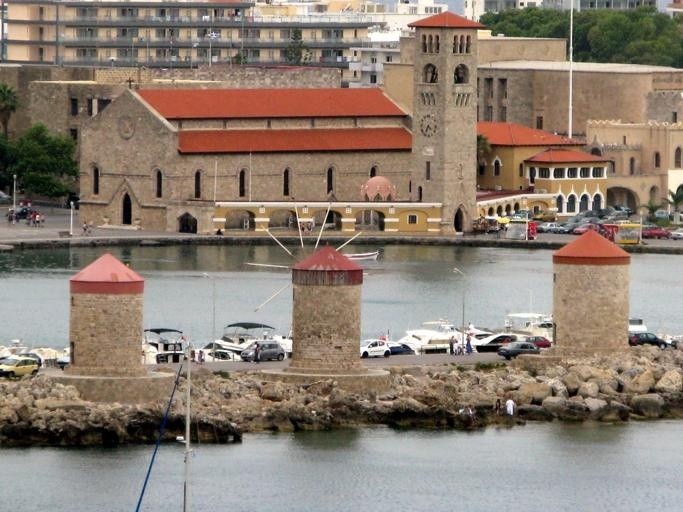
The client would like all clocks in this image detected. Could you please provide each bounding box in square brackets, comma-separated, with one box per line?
[419, 114, 437, 137]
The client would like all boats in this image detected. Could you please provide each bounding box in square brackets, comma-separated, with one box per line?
[0, 339, 71, 369]
[653, 332, 683, 350]
[627, 317, 648, 337]
[141, 322, 294, 366]
[340, 249, 384, 260]
[377, 312, 554, 354]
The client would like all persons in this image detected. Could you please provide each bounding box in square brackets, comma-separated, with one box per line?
[461, 405, 476, 422]
[12, 210, 16, 224]
[467, 404, 479, 421]
[505, 394, 515, 418]
[81, 221, 88, 236]
[452, 339, 465, 355]
[495, 398, 501, 416]
[26, 210, 45, 230]
[449, 335, 455, 355]
[465, 333, 473, 357]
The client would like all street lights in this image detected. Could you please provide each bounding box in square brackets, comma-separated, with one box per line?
[13, 173, 17, 224]
[201, 271, 216, 363]
[68, 201, 73, 236]
[451, 267, 468, 355]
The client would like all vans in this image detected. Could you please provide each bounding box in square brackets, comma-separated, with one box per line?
[0, 355, 38, 379]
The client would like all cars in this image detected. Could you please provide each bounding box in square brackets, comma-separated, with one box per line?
[497, 342, 541, 360]
[0, 191, 11, 202]
[15, 207, 31, 220]
[508, 205, 682, 240]
[358, 339, 392, 358]
[629, 333, 668, 350]
